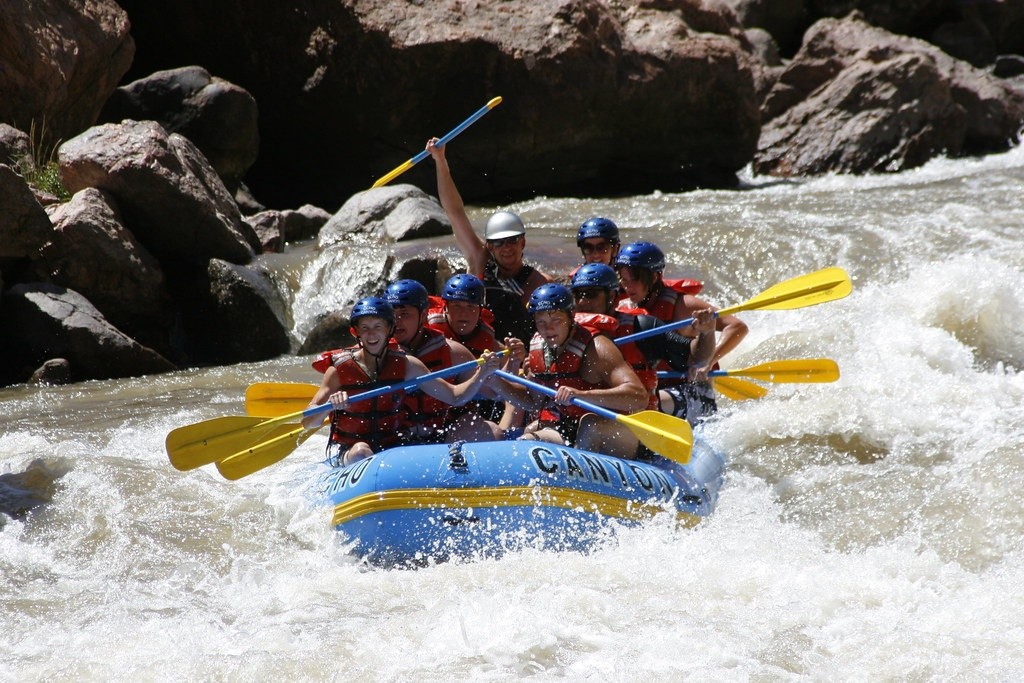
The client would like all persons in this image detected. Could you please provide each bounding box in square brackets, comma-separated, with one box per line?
[301, 138, 749, 468]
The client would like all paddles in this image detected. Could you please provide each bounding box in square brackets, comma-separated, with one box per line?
[359, 96, 502, 200]
[613, 267, 852, 347]
[165, 349, 511, 472]
[656, 358, 840, 384]
[215, 418, 331, 482]
[244, 382, 321, 418]
[710, 377, 769, 402]
[493, 369, 694, 465]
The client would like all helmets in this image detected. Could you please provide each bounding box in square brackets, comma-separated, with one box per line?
[350, 297, 395, 325]
[615, 240, 665, 272]
[383, 279, 430, 310]
[485, 212, 525, 240]
[442, 274, 485, 305]
[527, 283, 574, 313]
[570, 262, 620, 289]
[576, 218, 619, 247]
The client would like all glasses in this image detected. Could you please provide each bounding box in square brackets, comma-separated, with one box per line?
[581, 241, 611, 255]
[493, 236, 523, 247]
[573, 288, 607, 300]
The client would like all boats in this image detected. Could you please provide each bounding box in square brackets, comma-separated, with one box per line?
[308, 406, 725, 568]
